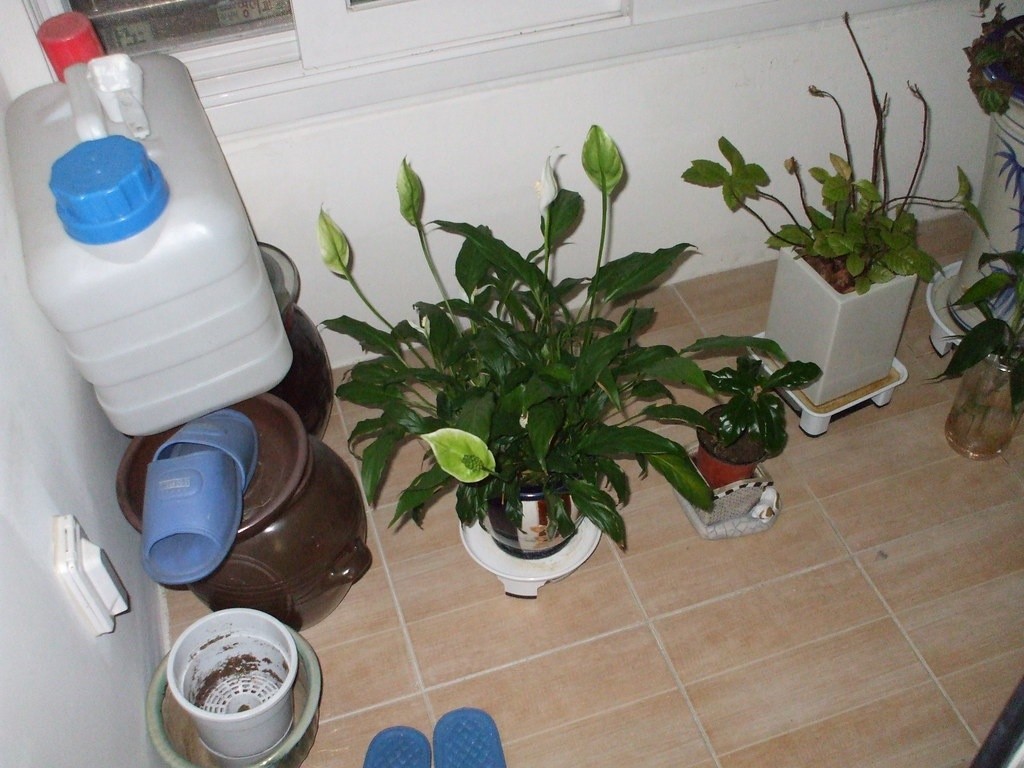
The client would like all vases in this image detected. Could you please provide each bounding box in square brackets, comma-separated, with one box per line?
[458, 503, 607, 602]
[145, 607, 323, 768]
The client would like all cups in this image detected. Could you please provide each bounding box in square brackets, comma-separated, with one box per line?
[36, 11, 106, 83]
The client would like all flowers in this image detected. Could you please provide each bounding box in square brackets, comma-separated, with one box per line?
[314, 126, 792, 553]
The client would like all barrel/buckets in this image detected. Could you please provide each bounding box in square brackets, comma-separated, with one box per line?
[6, 54, 294, 436]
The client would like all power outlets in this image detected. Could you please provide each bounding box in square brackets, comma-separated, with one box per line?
[53, 514, 133, 638]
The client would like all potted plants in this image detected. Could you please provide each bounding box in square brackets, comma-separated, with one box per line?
[680, 13, 991, 408]
[926, 249, 1024, 464]
[695, 357, 822, 489]
[948, 0, 1023, 350]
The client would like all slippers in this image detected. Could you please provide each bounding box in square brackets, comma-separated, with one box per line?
[141, 407, 258, 583]
[433, 706, 507, 768]
[363, 726, 432, 768]
